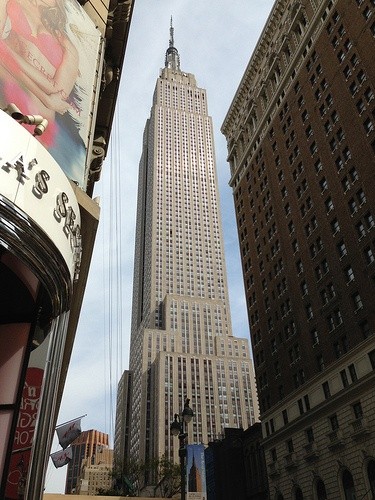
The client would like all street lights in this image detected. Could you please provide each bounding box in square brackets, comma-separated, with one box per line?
[170, 398, 194, 500]
